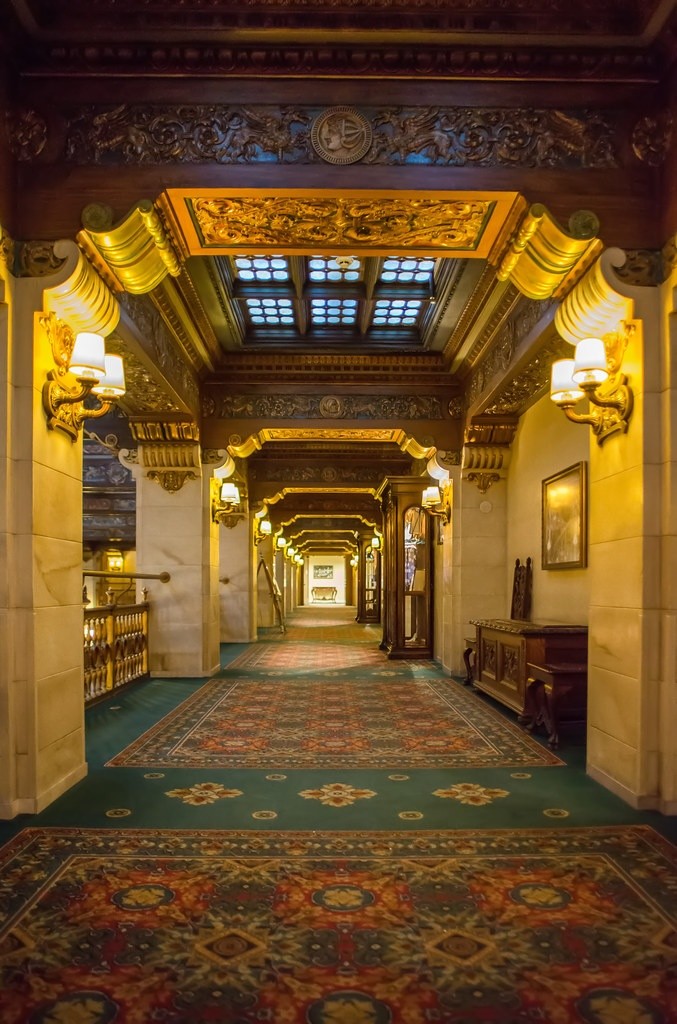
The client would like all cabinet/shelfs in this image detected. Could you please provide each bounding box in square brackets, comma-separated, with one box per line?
[471, 618, 587, 718]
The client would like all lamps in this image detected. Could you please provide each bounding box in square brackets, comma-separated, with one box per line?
[427, 486, 451, 521]
[422, 489, 447, 523]
[216, 487, 241, 522]
[212, 482, 235, 522]
[371, 538, 384, 553]
[44, 333, 106, 418]
[255, 520, 304, 567]
[571, 338, 622, 409]
[72, 355, 126, 429]
[548, 356, 603, 433]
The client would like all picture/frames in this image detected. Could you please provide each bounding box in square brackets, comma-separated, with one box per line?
[542, 461, 587, 571]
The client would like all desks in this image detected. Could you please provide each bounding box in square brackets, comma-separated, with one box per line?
[462, 638, 478, 678]
[524, 662, 587, 744]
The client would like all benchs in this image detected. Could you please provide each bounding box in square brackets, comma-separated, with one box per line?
[311, 587, 337, 604]
